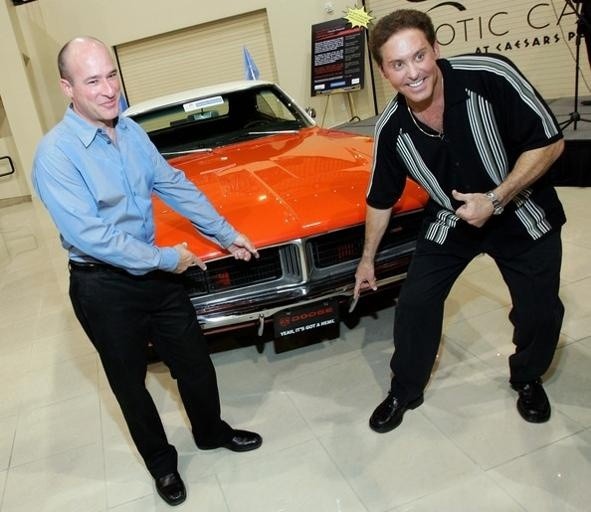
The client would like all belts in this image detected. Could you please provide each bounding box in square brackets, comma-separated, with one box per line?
[69, 259, 105, 268]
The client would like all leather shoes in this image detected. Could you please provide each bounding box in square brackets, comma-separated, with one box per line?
[510, 383, 551, 423]
[197, 429, 263, 452]
[155, 473, 187, 506]
[369, 391, 424, 433]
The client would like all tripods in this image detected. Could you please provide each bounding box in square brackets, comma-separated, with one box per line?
[558, 25, 591, 131]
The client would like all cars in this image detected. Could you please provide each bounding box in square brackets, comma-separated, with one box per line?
[116, 79, 436, 352]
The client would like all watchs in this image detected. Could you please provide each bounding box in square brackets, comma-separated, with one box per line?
[486, 189, 504, 216]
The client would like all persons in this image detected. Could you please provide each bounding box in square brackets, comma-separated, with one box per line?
[353, 8, 568, 433]
[32, 36, 263, 506]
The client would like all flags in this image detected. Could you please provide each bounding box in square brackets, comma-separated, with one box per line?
[242, 44, 260, 80]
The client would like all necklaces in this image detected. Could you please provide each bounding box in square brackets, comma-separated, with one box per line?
[406, 98, 444, 137]
